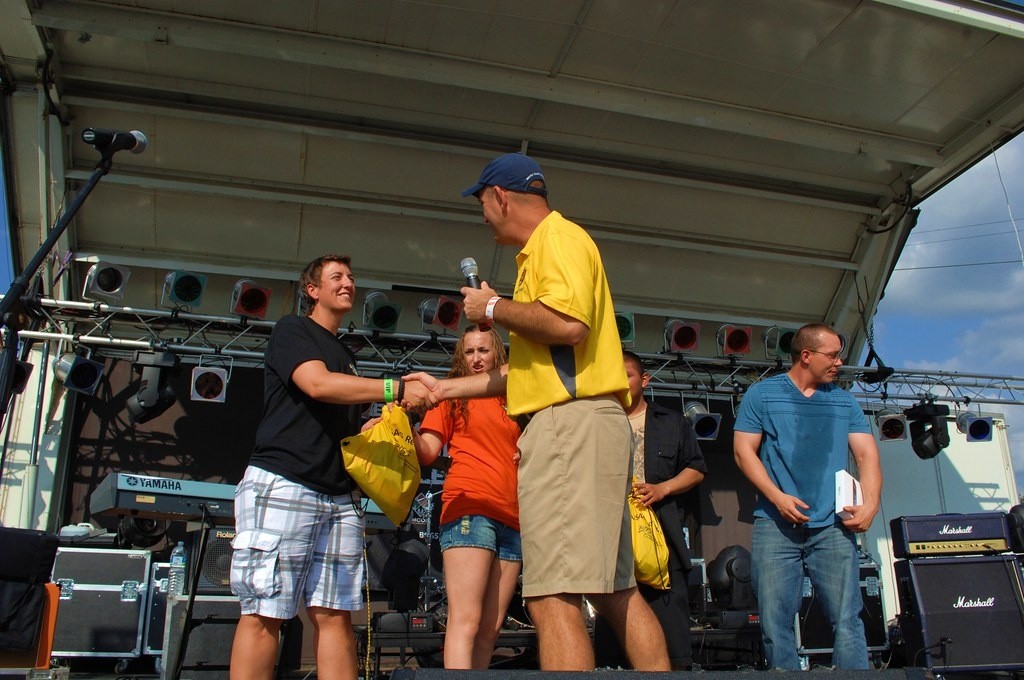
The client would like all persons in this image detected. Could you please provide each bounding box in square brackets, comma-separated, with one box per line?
[594, 351, 723, 671]
[733, 324, 882, 670]
[387, 321, 530, 670]
[230, 253, 439, 680]
[400, 152, 672, 671]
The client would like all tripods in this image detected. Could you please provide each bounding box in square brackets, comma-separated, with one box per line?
[416, 490, 448, 614]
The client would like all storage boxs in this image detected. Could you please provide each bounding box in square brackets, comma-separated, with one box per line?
[47, 546, 151, 672]
[143, 561, 169, 673]
[835, 470, 864, 521]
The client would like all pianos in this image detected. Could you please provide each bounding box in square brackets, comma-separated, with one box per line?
[88, 471, 414, 680]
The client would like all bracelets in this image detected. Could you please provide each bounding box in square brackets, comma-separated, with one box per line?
[384, 378, 394, 403]
[485, 296, 502, 323]
[398, 380, 405, 402]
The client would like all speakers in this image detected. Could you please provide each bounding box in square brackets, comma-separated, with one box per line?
[894, 554, 1024, 670]
[187, 524, 240, 597]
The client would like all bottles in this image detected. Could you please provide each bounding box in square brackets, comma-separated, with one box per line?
[168, 541, 187, 596]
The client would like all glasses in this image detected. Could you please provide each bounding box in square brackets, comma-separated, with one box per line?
[800, 349, 837, 364]
[465, 325, 476, 332]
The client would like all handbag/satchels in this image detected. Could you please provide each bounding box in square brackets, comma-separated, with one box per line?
[625, 474, 671, 589]
[339, 403, 421, 528]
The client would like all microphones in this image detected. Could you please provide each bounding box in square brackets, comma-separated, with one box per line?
[939, 635, 952, 643]
[81, 127, 148, 154]
[461, 257, 492, 332]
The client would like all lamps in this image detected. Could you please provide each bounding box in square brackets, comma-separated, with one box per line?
[613, 310, 803, 442]
[869, 401, 993, 460]
[13, 254, 464, 422]
[381, 541, 430, 597]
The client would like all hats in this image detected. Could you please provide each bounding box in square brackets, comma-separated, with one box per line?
[460, 152, 548, 199]
